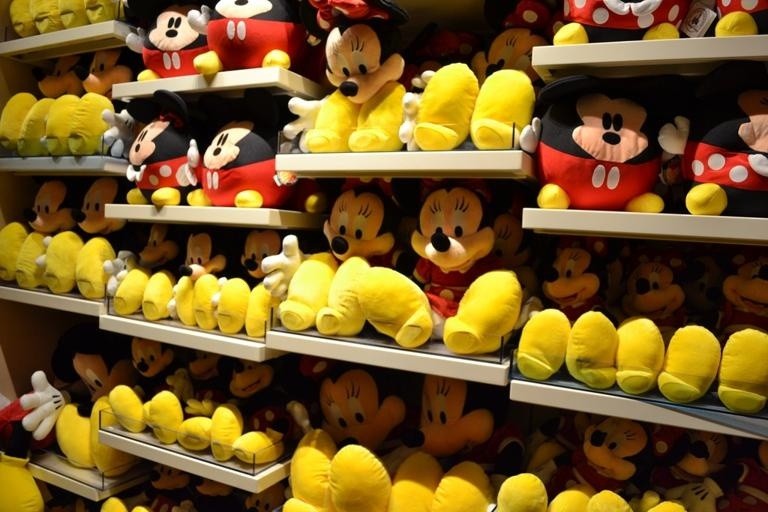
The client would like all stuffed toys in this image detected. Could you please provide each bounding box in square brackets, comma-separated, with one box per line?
[0, 0, 767, 512]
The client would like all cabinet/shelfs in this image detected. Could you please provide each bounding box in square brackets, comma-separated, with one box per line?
[0, 0, 768, 512]
